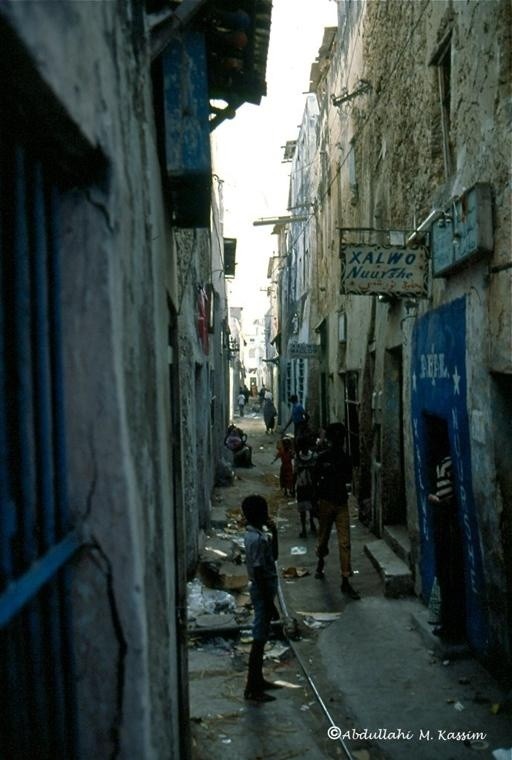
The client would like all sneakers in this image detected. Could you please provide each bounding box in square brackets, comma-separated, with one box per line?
[340, 582, 361, 601]
[316, 558, 326, 574]
[245, 680, 284, 703]
[310, 522, 317, 532]
[299, 529, 307, 538]
[427, 616, 464, 636]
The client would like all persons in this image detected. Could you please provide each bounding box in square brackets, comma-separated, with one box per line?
[294, 439, 321, 537]
[313, 422, 363, 600]
[260, 384, 266, 406]
[239, 386, 243, 394]
[236, 390, 245, 417]
[240, 493, 282, 704]
[263, 398, 277, 435]
[270, 438, 296, 498]
[282, 393, 311, 448]
[243, 385, 250, 404]
[431, 455, 465, 641]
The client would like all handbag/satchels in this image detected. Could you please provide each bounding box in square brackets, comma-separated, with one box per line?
[268, 417, 275, 428]
[276, 438, 284, 449]
[311, 503, 320, 519]
[294, 426, 313, 449]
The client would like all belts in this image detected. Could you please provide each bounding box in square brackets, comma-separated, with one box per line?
[320, 495, 348, 501]
